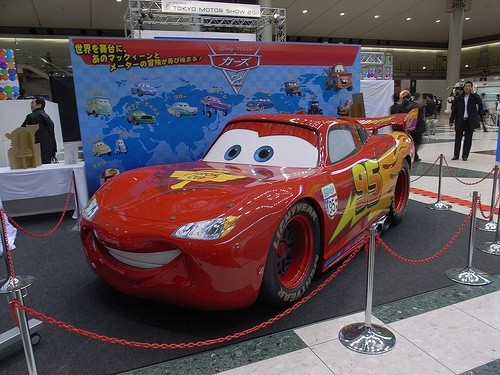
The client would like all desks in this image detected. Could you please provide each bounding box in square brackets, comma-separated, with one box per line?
[0, 161, 88, 218]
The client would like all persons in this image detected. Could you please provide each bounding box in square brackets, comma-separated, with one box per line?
[390, 90, 442, 163]
[18, 97, 57, 165]
[447, 81, 500, 161]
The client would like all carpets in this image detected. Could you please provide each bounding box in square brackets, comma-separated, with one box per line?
[0, 199, 500, 375]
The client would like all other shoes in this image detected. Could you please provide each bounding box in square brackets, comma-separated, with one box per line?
[452, 156, 459, 160]
[463, 156, 467, 160]
[414, 158, 421, 162]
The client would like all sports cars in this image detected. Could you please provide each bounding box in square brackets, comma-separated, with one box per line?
[77, 107, 420, 310]
